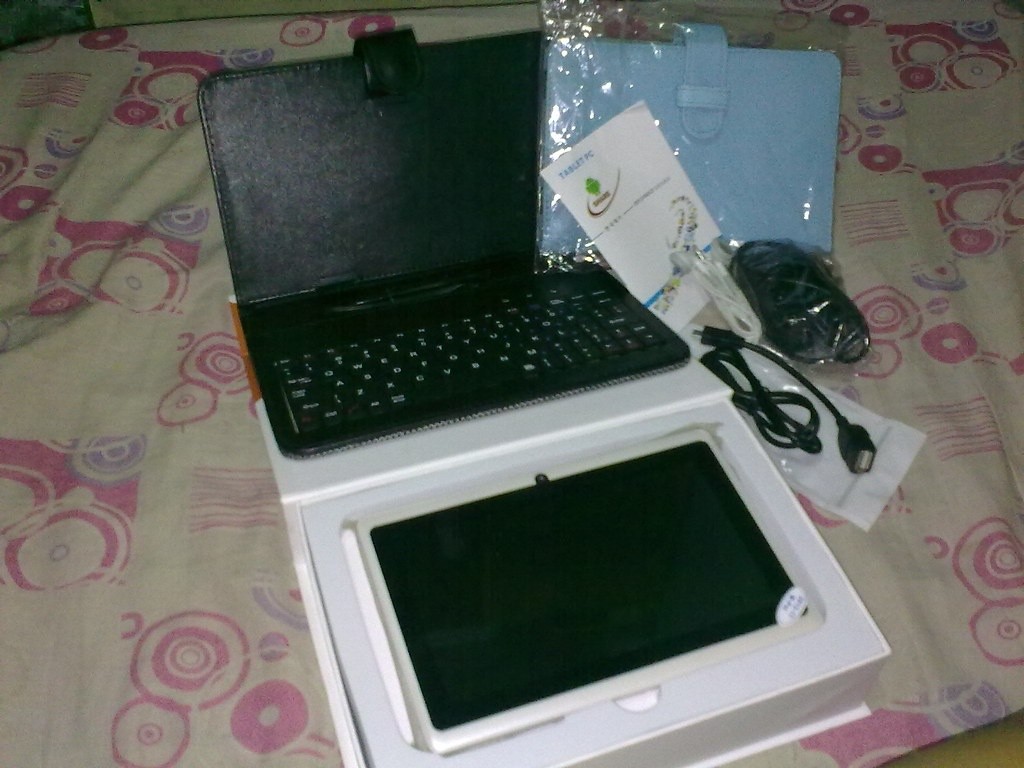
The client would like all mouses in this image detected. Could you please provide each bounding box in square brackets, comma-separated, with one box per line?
[728, 239, 871, 364]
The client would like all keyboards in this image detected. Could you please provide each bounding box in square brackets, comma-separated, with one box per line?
[239, 253, 692, 462]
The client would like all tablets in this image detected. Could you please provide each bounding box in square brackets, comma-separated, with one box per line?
[370, 439, 809, 731]
